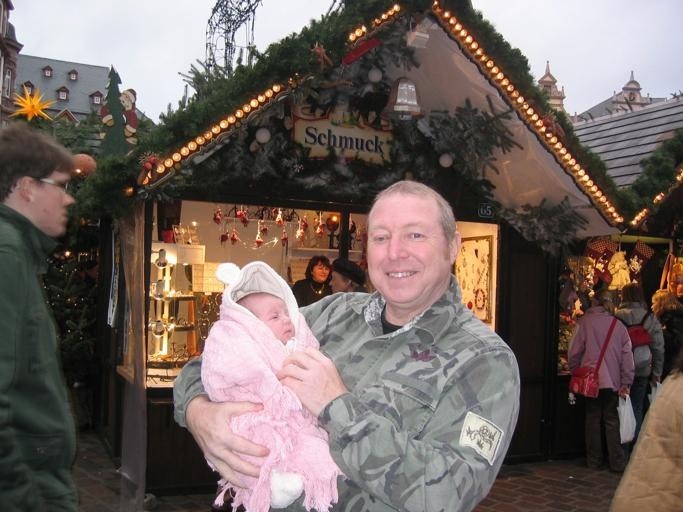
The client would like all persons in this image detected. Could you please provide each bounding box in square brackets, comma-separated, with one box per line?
[329, 259, 367, 293]
[171, 179, 524, 512]
[0, 125, 75, 512]
[199, 291, 345, 508]
[292, 254, 332, 307]
[567, 282, 682, 512]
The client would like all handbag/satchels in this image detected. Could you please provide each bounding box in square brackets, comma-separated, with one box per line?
[566, 365, 601, 401]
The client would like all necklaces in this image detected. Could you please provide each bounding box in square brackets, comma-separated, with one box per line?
[309, 283, 326, 295]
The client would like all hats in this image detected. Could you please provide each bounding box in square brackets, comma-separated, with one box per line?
[330, 257, 367, 286]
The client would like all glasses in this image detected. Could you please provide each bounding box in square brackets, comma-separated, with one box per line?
[8, 173, 71, 197]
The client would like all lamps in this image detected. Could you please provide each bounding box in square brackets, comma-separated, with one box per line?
[152, 248, 168, 269]
[152, 281, 167, 302]
[152, 322, 166, 339]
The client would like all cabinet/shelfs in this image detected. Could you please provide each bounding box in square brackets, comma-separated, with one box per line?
[553, 374, 585, 460]
[147, 295, 206, 369]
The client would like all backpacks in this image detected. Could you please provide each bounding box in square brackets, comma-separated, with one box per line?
[611, 310, 655, 350]
